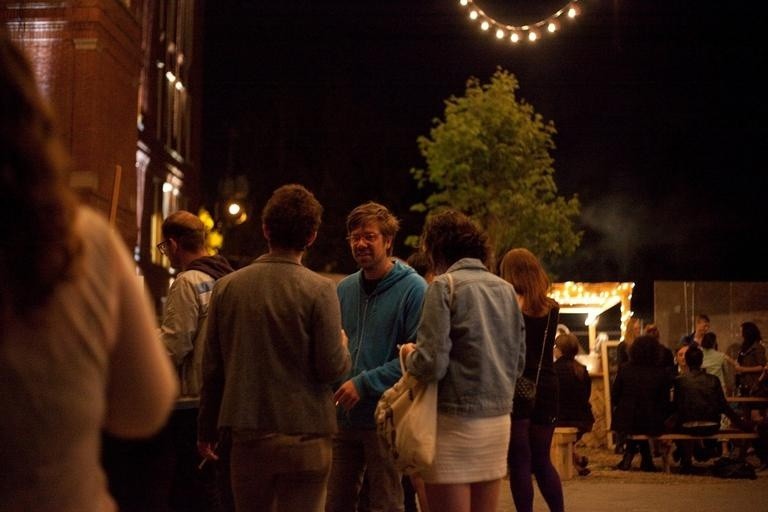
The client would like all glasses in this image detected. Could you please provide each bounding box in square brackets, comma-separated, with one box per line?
[457, 0, 582, 45]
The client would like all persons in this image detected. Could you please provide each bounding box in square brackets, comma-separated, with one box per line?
[552, 331, 596, 476]
[396, 207, 530, 511]
[156, 209, 236, 511]
[321, 201, 429, 511]
[0, 27, 181, 512]
[609, 313, 768, 477]
[195, 184, 353, 511]
[499, 248, 568, 512]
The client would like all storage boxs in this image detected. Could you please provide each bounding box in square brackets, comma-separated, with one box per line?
[626, 429, 758, 472]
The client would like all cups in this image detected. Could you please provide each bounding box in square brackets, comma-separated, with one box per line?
[157, 240, 172, 255]
[346, 234, 380, 243]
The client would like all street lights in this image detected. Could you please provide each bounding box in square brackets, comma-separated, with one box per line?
[374, 368, 438, 476]
[710, 456, 755, 479]
[513, 377, 536, 400]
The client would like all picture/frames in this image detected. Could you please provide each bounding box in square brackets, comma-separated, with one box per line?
[721, 395, 767, 425]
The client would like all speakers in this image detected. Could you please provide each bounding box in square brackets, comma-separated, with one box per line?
[618, 453, 633, 470]
[640, 455, 656, 472]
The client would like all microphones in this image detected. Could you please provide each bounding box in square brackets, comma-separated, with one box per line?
[545, 426, 577, 477]
